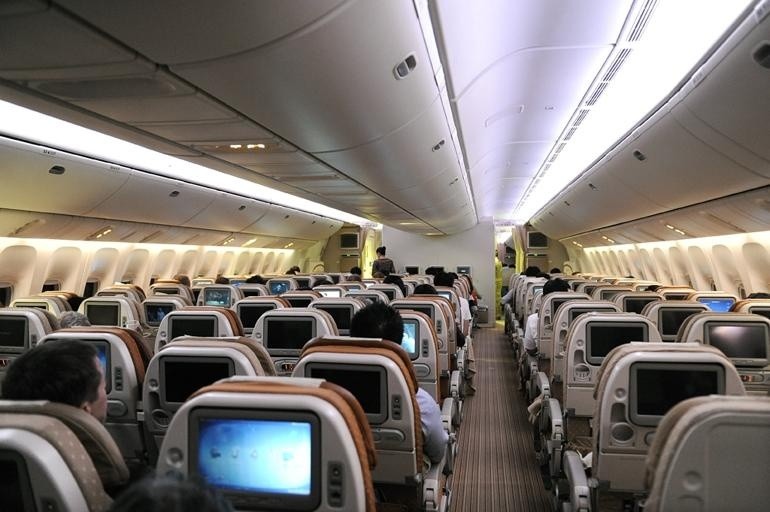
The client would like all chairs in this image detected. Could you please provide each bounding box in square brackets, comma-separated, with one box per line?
[0, 267, 478, 512]
[505, 270, 770, 512]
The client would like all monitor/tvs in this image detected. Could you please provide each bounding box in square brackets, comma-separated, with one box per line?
[269, 280, 290, 295]
[237, 302, 276, 333]
[630, 361, 727, 426]
[571, 310, 584, 319]
[704, 323, 770, 366]
[392, 305, 432, 317]
[230, 280, 246, 286]
[698, 297, 736, 314]
[204, 287, 231, 310]
[263, 316, 318, 356]
[307, 362, 388, 425]
[158, 356, 236, 409]
[381, 289, 396, 299]
[527, 231, 550, 249]
[287, 298, 314, 309]
[316, 289, 341, 298]
[339, 232, 359, 249]
[624, 296, 660, 313]
[406, 266, 418, 273]
[586, 322, 649, 364]
[295, 279, 309, 286]
[188, 405, 322, 511]
[0, 315, 29, 352]
[749, 307, 770, 319]
[604, 290, 615, 299]
[658, 307, 704, 340]
[665, 293, 692, 302]
[243, 289, 260, 297]
[144, 303, 175, 326]
[84, 301, 123, 329]
[168, 314, 219, 338]
[401, 317, 422, 362]
[552, 297, 568, 313]
[314, 305, 353, 334]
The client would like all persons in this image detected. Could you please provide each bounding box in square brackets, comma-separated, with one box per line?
[525, 278, 571, 357]
[276, 283, 282, 293]
[434, 272, 471, 336]
[351, 302, 446, 464]
[215, 277, 229, 284]
[216, 292, 222, 304]
[372, 246, 395, 278]
[500, 266, 540, 311]
[157, 308, 166, 322]
[0, 339, 106, 425]
[58, 311, 90, 328]
[174, 274, 190, 287]
[383, 275, 406, 301]
[402, 331, 414, 352]
[244, 275, 268, 297]
[351, 267, 361, 275]
[463, 275, 482, 309]
